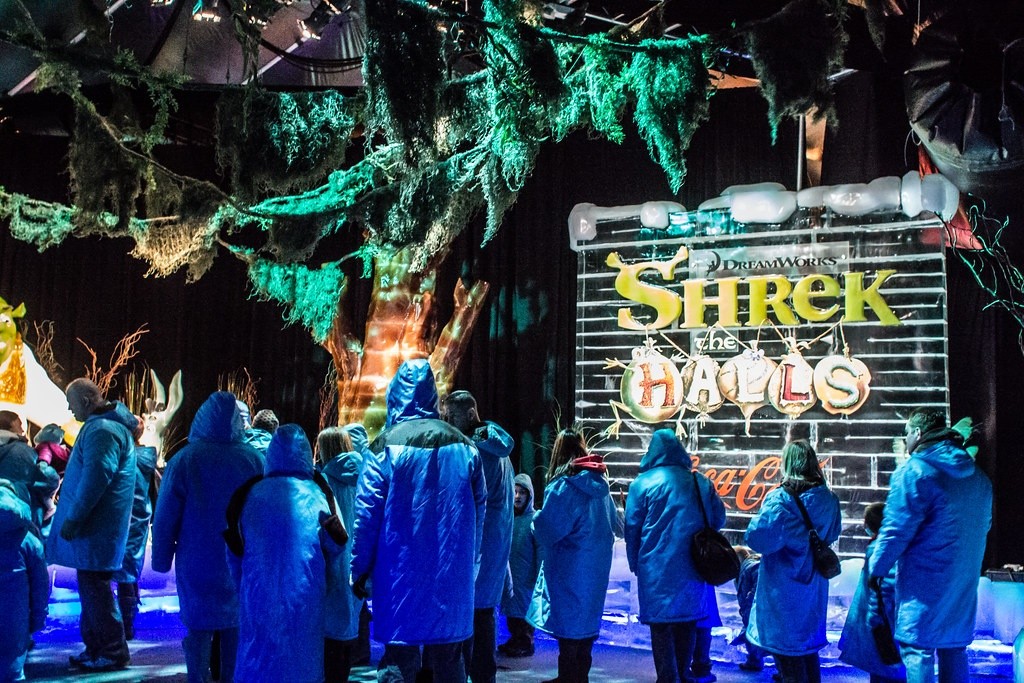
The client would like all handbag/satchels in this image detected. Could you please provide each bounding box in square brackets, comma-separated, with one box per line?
[693, 529, 741, 586]
[807, 526, 842, 580]
[872, 624, 903, 665]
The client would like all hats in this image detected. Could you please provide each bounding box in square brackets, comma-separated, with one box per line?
[34, 423, 65, 444]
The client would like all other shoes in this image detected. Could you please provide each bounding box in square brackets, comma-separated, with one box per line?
[497, 636, 536, 657]
[738, 659, 765, 672]
[69, 648, 127, 672]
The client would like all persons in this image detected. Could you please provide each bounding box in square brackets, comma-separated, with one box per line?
[867, 413, 993, 683]
[314, 359, 515, 683]
[625, 428, 726, 683]
[744, 440, 841, 683]
[838, 504, 907, 683]
[227, 425, 348, 683]
[525, 429, 621, 682]
[735, 547, 770, 670]
[498, 474, 537, 656]
[151, 392, 278, 683]
[0, 380, 157, 683]
